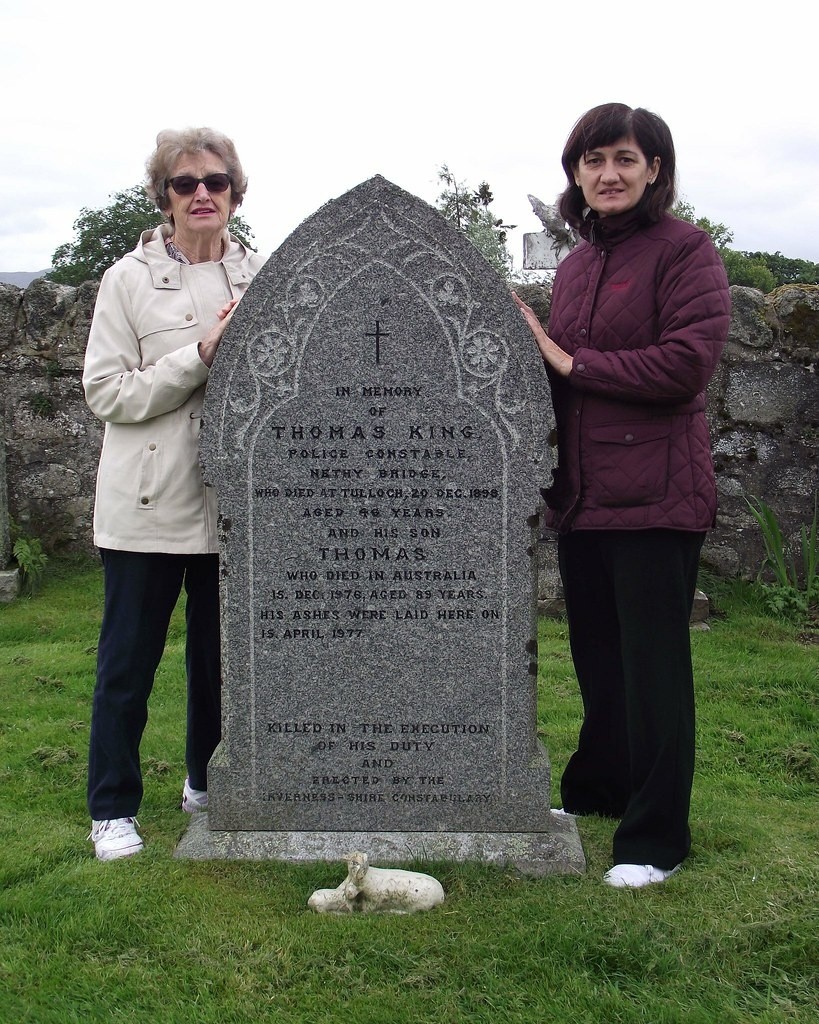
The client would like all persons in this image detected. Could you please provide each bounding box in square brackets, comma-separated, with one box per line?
[82, 127, 270, 861]
[511, 102, 731, 889]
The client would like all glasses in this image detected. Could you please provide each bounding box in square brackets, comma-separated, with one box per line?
[166, 173, 231, 195]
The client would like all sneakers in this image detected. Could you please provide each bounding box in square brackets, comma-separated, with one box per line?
[86, 817, 144, 861]
[550, 807, 582, 818]
[602, 864, 681, 888]
[181, 779, 208, 813]
